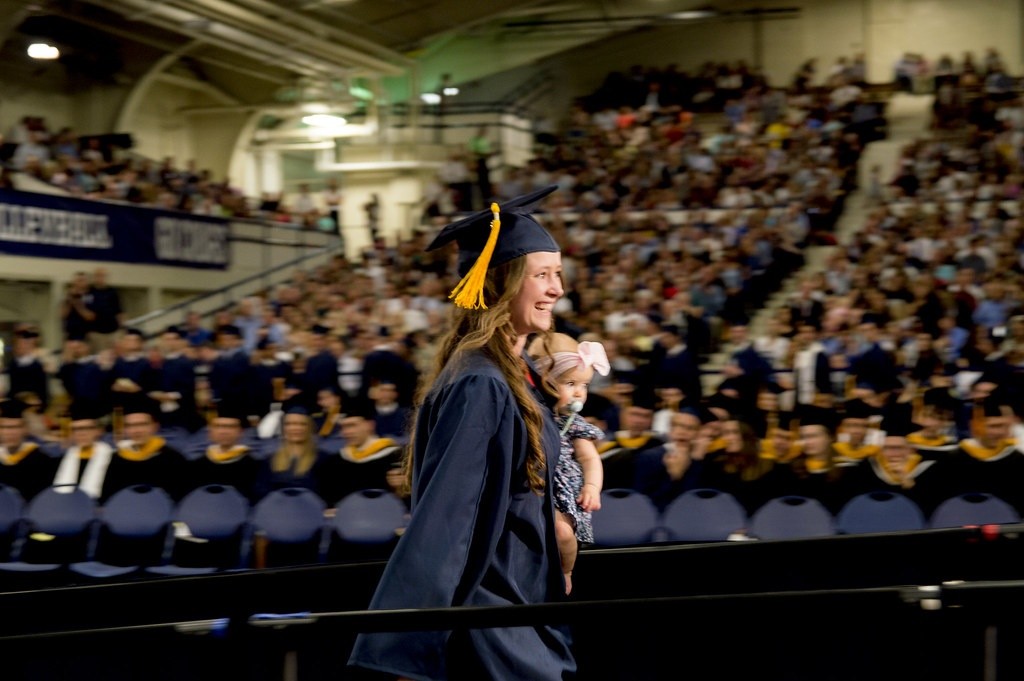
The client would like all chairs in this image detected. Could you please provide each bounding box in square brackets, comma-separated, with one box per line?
[68, 485, 177, 583]
[930, 492, 1021, 529]
[222, 487, 325, 574]
[0, 484, 98, 579]
[0, 482, 26, 541]
[658, 488, 747, 543]
[591, 487, 660, 546]
[750, 494, 835, 540]
[838, 491, 925, 533]
[146, 483, 251, 577]
[326, 488, 406, 562]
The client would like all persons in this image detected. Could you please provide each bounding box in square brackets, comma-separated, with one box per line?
[526, 332, 610, 596]
[345, 185, 577, 681]
[0, 47, 1024, 566]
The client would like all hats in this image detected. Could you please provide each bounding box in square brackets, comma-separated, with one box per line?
[0, 310, 1024, 458]
[424, 185, 559, 311]
